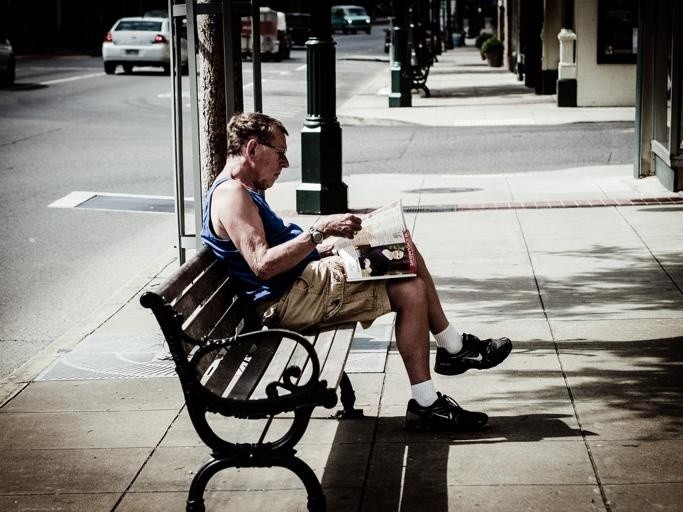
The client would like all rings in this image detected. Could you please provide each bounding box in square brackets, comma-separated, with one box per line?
[352, 230, 356, 236]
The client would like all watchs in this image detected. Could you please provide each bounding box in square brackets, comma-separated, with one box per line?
[309, 226, 328, 246]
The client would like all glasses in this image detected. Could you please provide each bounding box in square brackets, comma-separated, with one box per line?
[256, 140, 288, 157]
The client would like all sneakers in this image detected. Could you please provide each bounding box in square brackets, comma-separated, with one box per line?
[404, 390, 489, 435]
[434, 333, 513, 375]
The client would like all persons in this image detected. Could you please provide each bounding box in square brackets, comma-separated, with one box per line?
[357, 240, 410, 275]
[201, 111, 517, 431]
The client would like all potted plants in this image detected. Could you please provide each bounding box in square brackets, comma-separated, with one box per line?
[475, 31, 505, 68]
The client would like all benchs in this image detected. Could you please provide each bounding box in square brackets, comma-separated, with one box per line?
[141, 245, 369, 512]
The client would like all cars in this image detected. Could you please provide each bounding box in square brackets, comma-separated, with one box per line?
[99, 5, 373, 81]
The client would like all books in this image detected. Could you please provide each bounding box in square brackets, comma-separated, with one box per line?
[331, 196, 418, 284]
